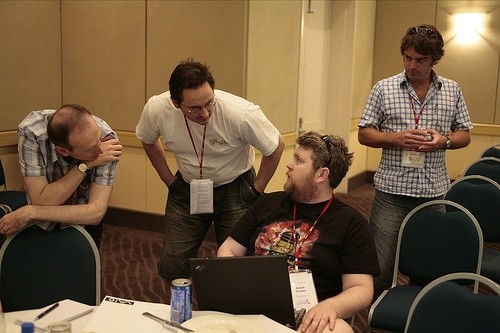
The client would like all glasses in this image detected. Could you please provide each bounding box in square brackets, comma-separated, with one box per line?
[406, 25, 438, 40]
[320, 135, 332, 166]
[179, 94, 218, 114]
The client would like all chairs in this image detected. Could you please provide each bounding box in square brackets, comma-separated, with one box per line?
[369, 144, 500, 333]
[0, 161, 101, 313]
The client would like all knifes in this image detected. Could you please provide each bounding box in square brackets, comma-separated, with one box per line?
[143, 312, 194, 333]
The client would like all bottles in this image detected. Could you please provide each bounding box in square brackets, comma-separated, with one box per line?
[22, 322, 35, 333]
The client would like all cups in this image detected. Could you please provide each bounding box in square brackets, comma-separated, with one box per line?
[50, 321, 71, 333]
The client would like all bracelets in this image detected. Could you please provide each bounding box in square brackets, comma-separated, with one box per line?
[250, 185, 262, 198]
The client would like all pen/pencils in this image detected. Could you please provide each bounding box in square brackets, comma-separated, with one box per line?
[34, 303, 59, 321]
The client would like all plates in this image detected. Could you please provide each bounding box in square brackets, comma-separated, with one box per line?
[177, 315, 250, 333]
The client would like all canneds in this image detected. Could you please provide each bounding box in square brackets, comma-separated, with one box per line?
[170, 279, 192, 322]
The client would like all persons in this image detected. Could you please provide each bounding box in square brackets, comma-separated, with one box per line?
[0, 102, 125, 248]
[356, 21, 474, 311]
[134, 57, 286, 281]
[215, 129, 382, 332]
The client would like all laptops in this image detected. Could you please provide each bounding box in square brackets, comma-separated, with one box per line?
[187, 256, 306, 331]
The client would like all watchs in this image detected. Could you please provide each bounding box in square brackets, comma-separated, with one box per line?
[75, 160, 90, 175]
[445, 136, 451, 150]
